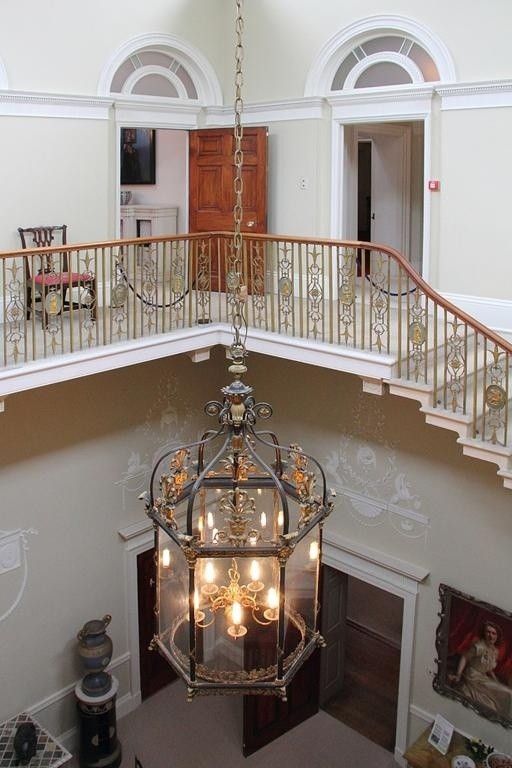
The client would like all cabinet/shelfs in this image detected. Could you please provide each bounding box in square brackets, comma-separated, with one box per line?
[120, 207, 179, 283]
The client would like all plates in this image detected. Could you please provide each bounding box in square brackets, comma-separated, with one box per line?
[450, 755, 476, 768]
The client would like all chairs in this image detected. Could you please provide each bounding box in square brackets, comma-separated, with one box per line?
[17, 224, 96, 328]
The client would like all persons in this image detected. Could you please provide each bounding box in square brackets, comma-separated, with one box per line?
[121, 128, 142, 183]
[446, 618, 511, 723]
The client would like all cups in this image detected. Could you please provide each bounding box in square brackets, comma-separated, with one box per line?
[120, 191, 133, 205]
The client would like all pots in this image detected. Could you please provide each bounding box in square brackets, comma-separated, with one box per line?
[482, 752, 512, 768]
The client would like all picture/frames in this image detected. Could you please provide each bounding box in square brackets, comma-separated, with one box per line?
[120, 127, 156, 185]
[432, 582, 511, 729]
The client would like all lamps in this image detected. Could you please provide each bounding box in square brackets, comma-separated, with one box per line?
[137, 0, 336, 703]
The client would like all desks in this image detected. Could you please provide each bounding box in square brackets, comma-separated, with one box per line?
[400, 718, 512, 767]
